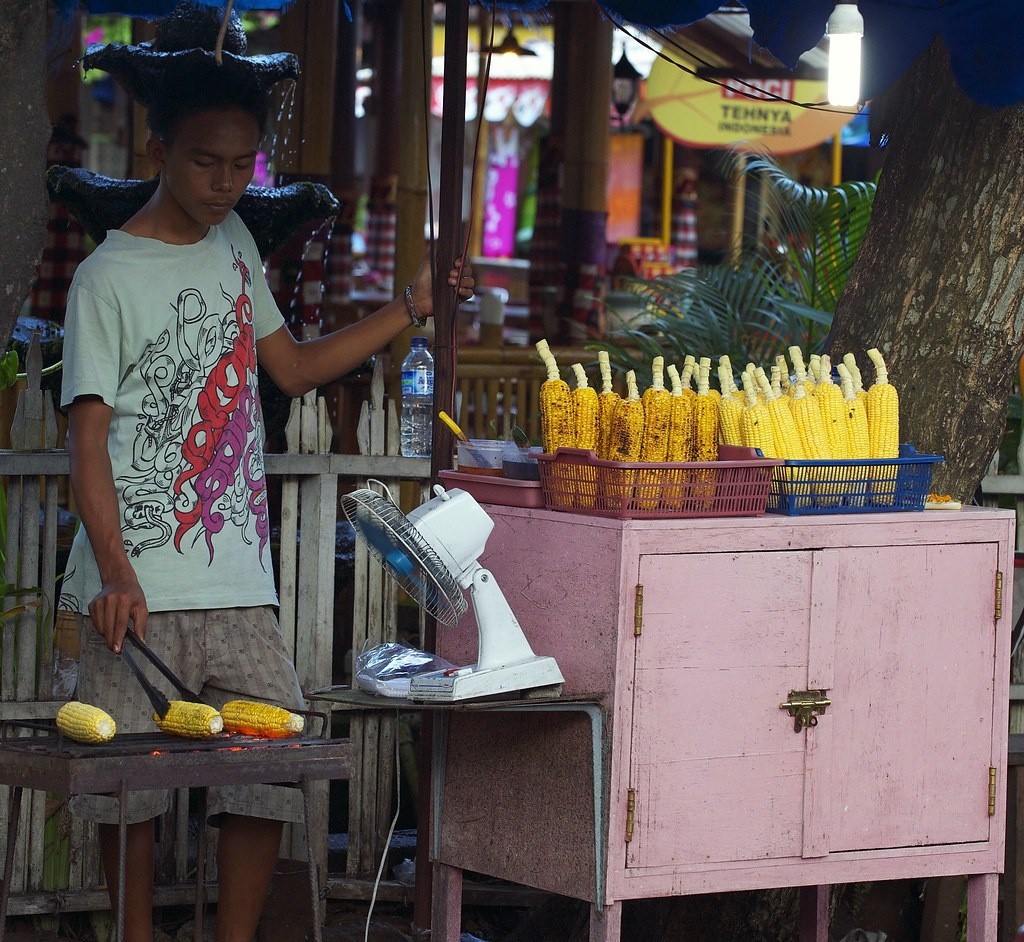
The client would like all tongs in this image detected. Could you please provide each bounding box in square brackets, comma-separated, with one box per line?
[119, 627, 204, 722]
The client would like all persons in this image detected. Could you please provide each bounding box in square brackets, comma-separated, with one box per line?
[350, 95, 383, 179]
[60, 75, 476, 942]
[607, 244, 638, 276]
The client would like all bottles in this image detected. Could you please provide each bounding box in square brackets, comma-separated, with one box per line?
[400, 338, 433, 456]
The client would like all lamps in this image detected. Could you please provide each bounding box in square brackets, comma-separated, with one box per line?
[480, 22, 536, 56]
[610, 42, 644, 130]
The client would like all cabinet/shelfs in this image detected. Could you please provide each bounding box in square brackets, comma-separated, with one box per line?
[428, 498, 1015, 942]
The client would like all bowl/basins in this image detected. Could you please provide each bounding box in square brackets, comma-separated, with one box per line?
[456, 438, 504, 477]
[502, 447, 543, 481]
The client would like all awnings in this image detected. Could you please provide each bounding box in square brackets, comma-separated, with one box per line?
[431, 25, 661, 127]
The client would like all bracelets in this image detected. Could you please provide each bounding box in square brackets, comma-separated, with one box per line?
[399, 283, 427, 329]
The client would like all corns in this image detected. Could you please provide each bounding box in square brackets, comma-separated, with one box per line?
[538, 381, 899, 507]
[152, 701, 306, 738]
[57, 702, 117, 743]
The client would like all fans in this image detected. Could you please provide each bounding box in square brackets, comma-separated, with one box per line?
[341, 479, 565, 702]
